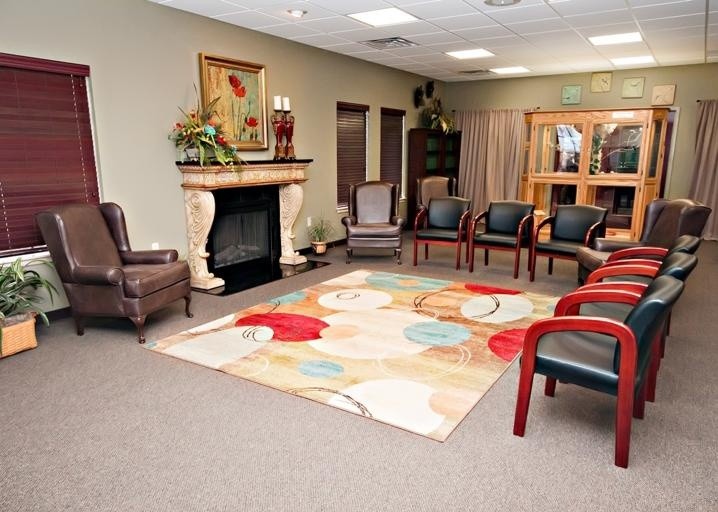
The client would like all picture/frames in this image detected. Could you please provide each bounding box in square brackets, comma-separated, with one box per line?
[199, 51, 270, 151]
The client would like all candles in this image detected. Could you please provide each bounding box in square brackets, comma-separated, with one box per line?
[283, 97, 290, 111]
[273, 96, 281, 110]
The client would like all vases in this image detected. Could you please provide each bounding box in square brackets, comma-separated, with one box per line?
[282, 111, 296, 160]
[185, 149, 200, 162]
[271, 110, 286, 160]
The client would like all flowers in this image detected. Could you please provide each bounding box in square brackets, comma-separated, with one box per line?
[168, 83, 248, 166]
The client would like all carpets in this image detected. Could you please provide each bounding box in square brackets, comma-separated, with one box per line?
[144, 266, 565, 444]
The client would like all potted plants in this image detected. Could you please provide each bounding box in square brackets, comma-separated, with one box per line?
[414, 80, 457, 135]
[307, 208, 335, 256]
[0, 259, 60, 359]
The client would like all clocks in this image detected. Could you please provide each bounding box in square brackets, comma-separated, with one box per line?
[561, 72, 676, 107]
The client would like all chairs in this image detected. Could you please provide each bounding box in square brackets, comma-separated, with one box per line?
[529, 204, 608, 287]
[35, 202, 194, 345]
[468, 200, 536, 279]
[565, 252, 697, 402]
[513, 273, 686, 466]
[577, 198, 712, 289]
[341, 181, 407, 265]
[414, 196, 472, 271]
[596, 235, 702, 359]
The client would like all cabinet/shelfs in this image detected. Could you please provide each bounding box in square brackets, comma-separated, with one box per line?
[520, 107, 670, 244]
[404, 128, 462, 231]
